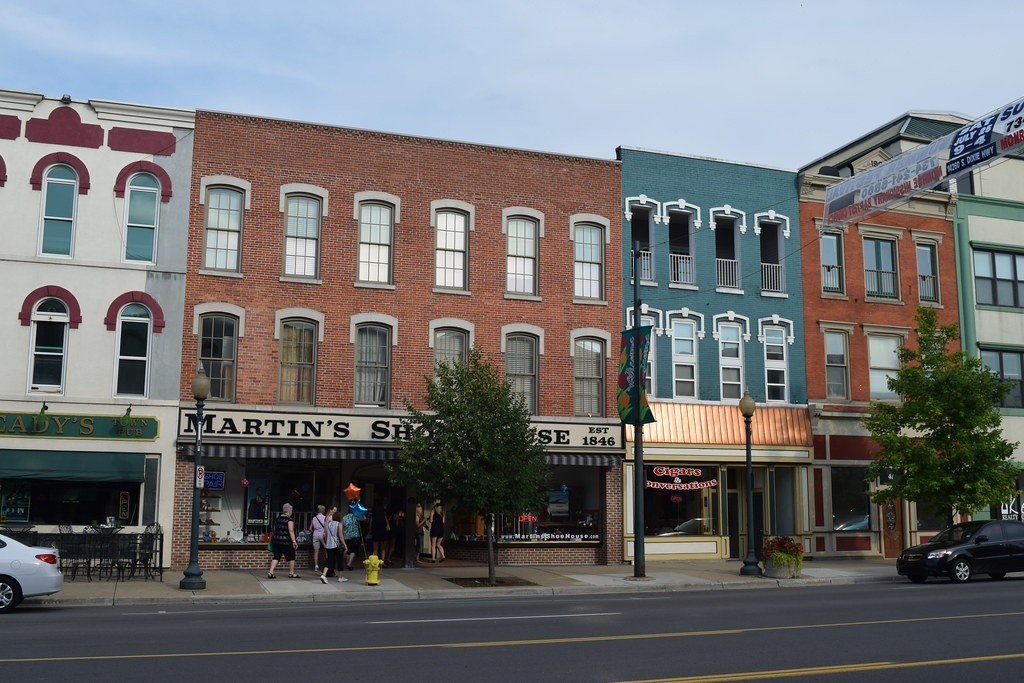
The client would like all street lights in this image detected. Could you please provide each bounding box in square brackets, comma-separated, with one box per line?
[739, 388, 762, 576]
[179, 366, 212, 589]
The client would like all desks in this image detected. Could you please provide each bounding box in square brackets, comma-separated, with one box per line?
[0, 525, 35, 540]
[87, 526, 126, 583]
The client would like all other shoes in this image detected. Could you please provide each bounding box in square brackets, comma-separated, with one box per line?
[346, 565, 354, 571]
[430, 560, 435, 563]
[439, 558, 445, 562]
[314, 565, 319, 571]
[338, 577, 348, 582]
[320, 575, 328, 584]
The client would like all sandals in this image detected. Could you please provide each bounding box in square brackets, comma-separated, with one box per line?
[288, 573, 301, 578]
[268, 572, 277, 578]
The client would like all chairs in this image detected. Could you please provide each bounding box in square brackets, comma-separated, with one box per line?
[58, 521, 161, 582]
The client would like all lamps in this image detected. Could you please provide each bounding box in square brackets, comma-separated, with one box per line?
[120, 403, 133, 423]
[60, 94, 72, 104]
[36, 401, 49, 422]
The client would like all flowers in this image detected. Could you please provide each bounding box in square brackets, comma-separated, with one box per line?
[760, 535, 804, 572]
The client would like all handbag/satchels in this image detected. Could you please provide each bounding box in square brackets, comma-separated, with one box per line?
[337, 542, 345, 556]
[268, 537, 273, 551]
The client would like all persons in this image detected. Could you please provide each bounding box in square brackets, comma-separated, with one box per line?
[248, 487, 265, 519]
[416, 506, 429, 563]
[428, 503, 447, 563]
[267, 503, 302, 579]
[309, 505, 399, 584]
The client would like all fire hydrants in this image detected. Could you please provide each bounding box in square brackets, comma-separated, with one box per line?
[363, 553, 384, 586]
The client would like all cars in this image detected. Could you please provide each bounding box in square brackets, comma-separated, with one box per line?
[0, 534, 64, 614]
[896, 518, 1024, 584]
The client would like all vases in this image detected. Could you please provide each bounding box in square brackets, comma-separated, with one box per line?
[765, 557, 801, 579]
